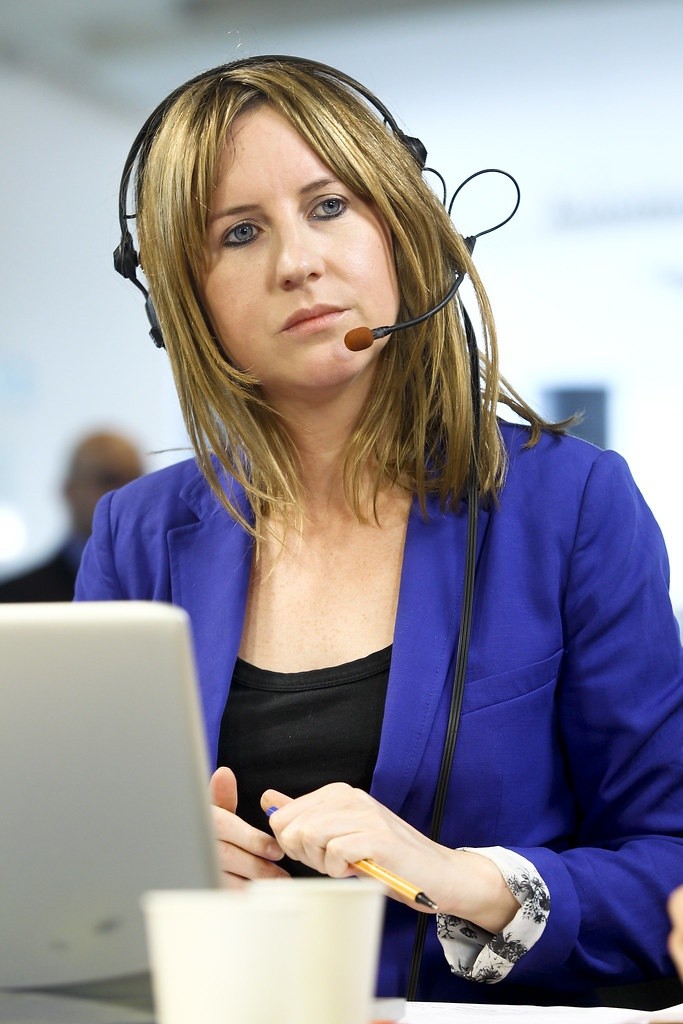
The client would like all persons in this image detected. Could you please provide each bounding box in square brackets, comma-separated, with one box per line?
[0, 429, 148, 602]
[74, 54, 683, 1024]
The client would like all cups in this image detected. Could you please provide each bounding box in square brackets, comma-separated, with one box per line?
[138, 877, 388, 1024]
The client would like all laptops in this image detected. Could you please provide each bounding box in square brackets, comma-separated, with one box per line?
[0, 600, 407, 1024]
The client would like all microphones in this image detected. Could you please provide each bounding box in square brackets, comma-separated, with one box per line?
[343, 237, 477, 352]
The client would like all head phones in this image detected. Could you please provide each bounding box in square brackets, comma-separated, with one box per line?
[112, 54, 464, 354]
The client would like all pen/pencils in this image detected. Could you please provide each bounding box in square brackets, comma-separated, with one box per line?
[268, 806, 441, 912]
